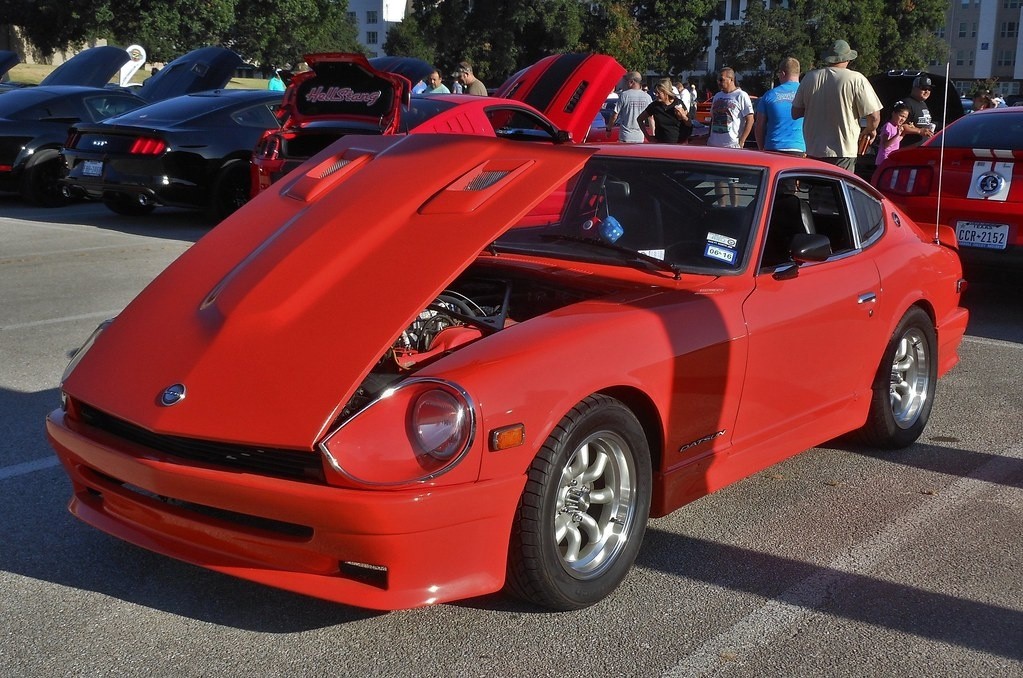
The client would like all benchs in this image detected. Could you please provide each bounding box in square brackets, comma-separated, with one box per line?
[709, 205, 847, 263]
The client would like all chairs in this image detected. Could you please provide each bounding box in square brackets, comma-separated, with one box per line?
[551, 190, 666, 259]
[697, 190, 817, 268]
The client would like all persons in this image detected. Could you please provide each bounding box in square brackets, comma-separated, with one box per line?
[873, 100, 915, 172]
[597, 70, 713, 146]
[704, 66, 754, 211]
[898, 76, 934, 148]
[142, 66, 159, 85]
[790, 39, 883, 175]
[755, 58, 807, 196]
[406, 62, 488, 97]
[267, 67, 286, 90]
[966, 88, 1008, 113]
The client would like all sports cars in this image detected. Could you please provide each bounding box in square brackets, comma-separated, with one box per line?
[252, 52, 626, 243]
[60, 56, 436, 216]
[0, 51, 32, 95]
[45, 134, 970, 614]
[587, 91, 762, 153]
[855, 69, 965, 184]
[0, 46, 139, 98]
[1, 46, 243, 207]
[960, 94, 1023, 113]
[869, 106, 1023, 282]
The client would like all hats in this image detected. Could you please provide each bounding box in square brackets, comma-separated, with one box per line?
[912, 76, 936, 88]
[820, 39, 858, 64]
